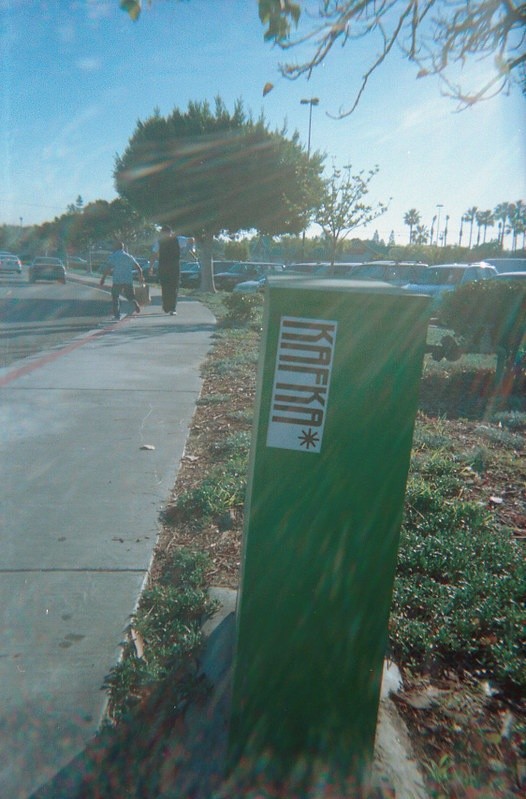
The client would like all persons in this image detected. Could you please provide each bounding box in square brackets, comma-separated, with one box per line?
[148, 225, 179, 314]
[100, 241, 144, 320]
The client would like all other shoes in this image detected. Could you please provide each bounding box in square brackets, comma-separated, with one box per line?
[112, 316, 120, 320]
[132, 300, 140, 313]
[165, 310, 176, 315]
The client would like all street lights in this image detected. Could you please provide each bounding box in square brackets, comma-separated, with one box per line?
[299, 97, 319, 260]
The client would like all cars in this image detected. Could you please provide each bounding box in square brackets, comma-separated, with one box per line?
[487, 272, 526, 285]
[0, 252, 22, 273]
[28, 256, 66, 284]
[134, 257, 199, 287]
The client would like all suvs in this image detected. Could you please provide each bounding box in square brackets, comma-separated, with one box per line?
[194, 260, 285, 298]
[287, 261, 496, 318]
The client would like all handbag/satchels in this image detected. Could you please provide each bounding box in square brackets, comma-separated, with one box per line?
[134, 282, 151, 304]
